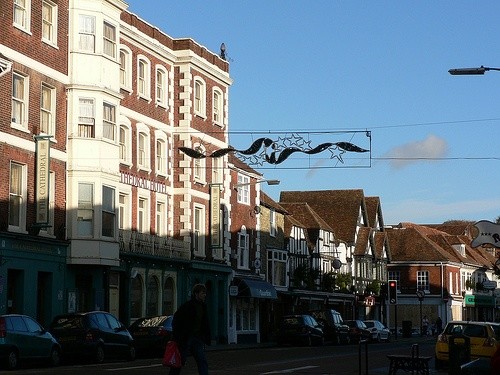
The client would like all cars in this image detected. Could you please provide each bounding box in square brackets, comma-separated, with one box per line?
[51, 310, 136, 362]
[129, 315, 176, 354]
[279, 314, 324, 342]
[434, 321, 498, 369]
[345, 319, 370, 341]
[364, 319, 392, 342]
[1, 314, 64, 366]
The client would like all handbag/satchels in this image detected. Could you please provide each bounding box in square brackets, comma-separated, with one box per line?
[162, 339, 183, 369]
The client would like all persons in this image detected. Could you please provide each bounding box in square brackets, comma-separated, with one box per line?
[171, 284, 211, 375]
[435, 317, 442, 336]
[421, 315, 429, 336]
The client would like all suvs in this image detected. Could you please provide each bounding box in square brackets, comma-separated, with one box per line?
[304, 310, 351, 344]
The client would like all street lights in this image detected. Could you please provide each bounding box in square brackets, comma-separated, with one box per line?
[235, 179, 281, 188]
[448, 65, 500, 75]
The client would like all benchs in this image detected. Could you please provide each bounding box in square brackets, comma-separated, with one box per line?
[386, 354, 433, 375]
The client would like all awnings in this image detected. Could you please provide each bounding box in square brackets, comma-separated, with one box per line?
[230, 279, 277, 301]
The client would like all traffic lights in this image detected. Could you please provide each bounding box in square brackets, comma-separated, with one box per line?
[387, 279, 398, 307]
